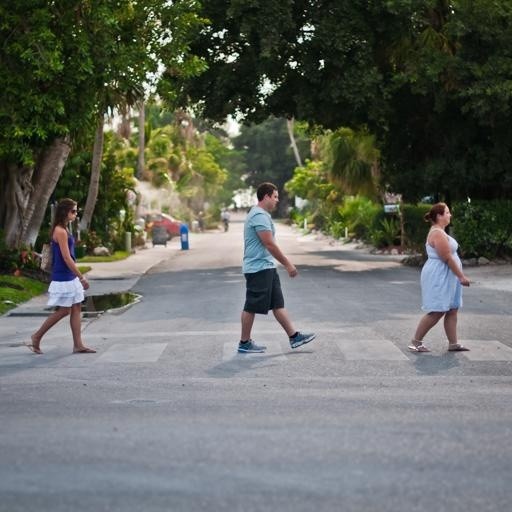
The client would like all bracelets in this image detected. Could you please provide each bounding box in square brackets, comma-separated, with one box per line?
[80, 276, 86, 283]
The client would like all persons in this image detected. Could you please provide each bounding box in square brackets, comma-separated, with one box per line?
[21, 196, 99, 355]
[220, 207, 230, 233]
[403, 201, 471, 353]
[191, 209, 206, 234]
[236, 182, 317, 354]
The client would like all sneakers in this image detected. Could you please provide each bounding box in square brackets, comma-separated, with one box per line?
[238, 339, 266, 353]
[290, 332, 316, 348]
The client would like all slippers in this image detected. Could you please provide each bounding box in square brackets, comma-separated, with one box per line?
[73, 347, 96, 353]
[22, 341, 43, 354]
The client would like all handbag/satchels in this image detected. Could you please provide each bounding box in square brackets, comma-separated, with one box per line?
[39, 244, 53, 273]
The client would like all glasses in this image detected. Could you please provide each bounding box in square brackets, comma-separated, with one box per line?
[71, 209, 78, 214]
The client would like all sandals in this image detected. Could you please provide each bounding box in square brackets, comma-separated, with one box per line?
[408, 340, 431, 352]
[448, 343, 470, 351]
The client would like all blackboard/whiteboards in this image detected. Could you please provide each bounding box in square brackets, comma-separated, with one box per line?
[152, 224, 167, 245]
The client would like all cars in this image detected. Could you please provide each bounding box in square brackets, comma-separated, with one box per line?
[143, 211, 185, 241]
